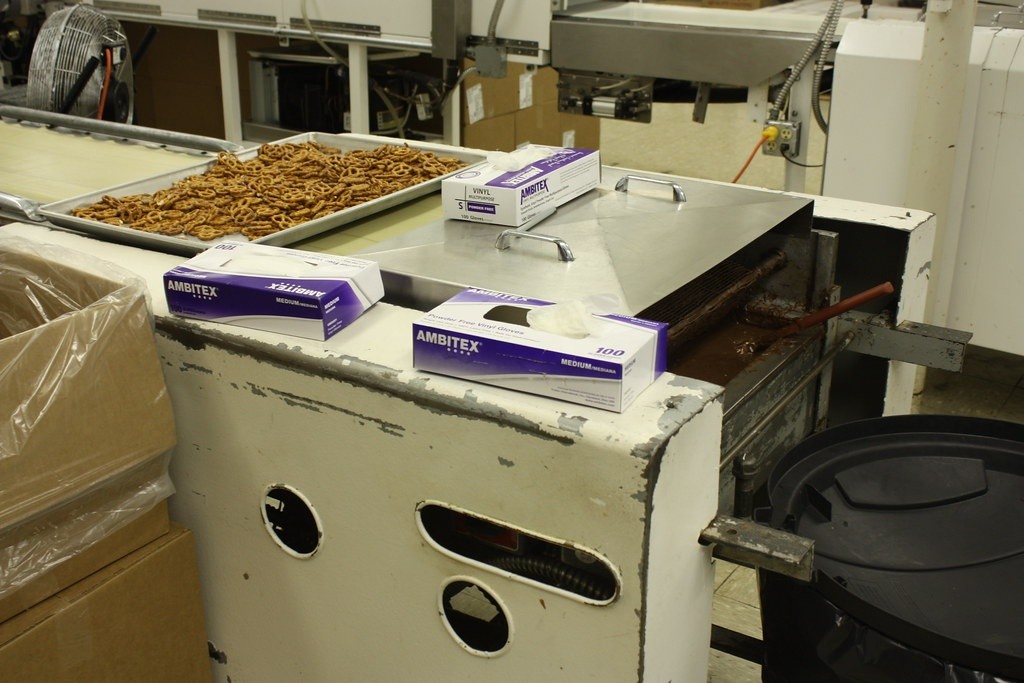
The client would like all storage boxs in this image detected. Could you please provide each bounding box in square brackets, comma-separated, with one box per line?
[0, 243, 177, 625]
[516, 99, 559, 152]
[460, 51, 559, 125]
[0, 521, 212, 683]
[559, 113, 601, 182]
[462, 112, 516, 158]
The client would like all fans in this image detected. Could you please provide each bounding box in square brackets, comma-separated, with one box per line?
[26, 3, 134, 125]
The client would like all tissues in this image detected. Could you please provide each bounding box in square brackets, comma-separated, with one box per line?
[163, 242, 386, 340]
[413, 291, 670, 412]
[441, 143, 601, 225]
